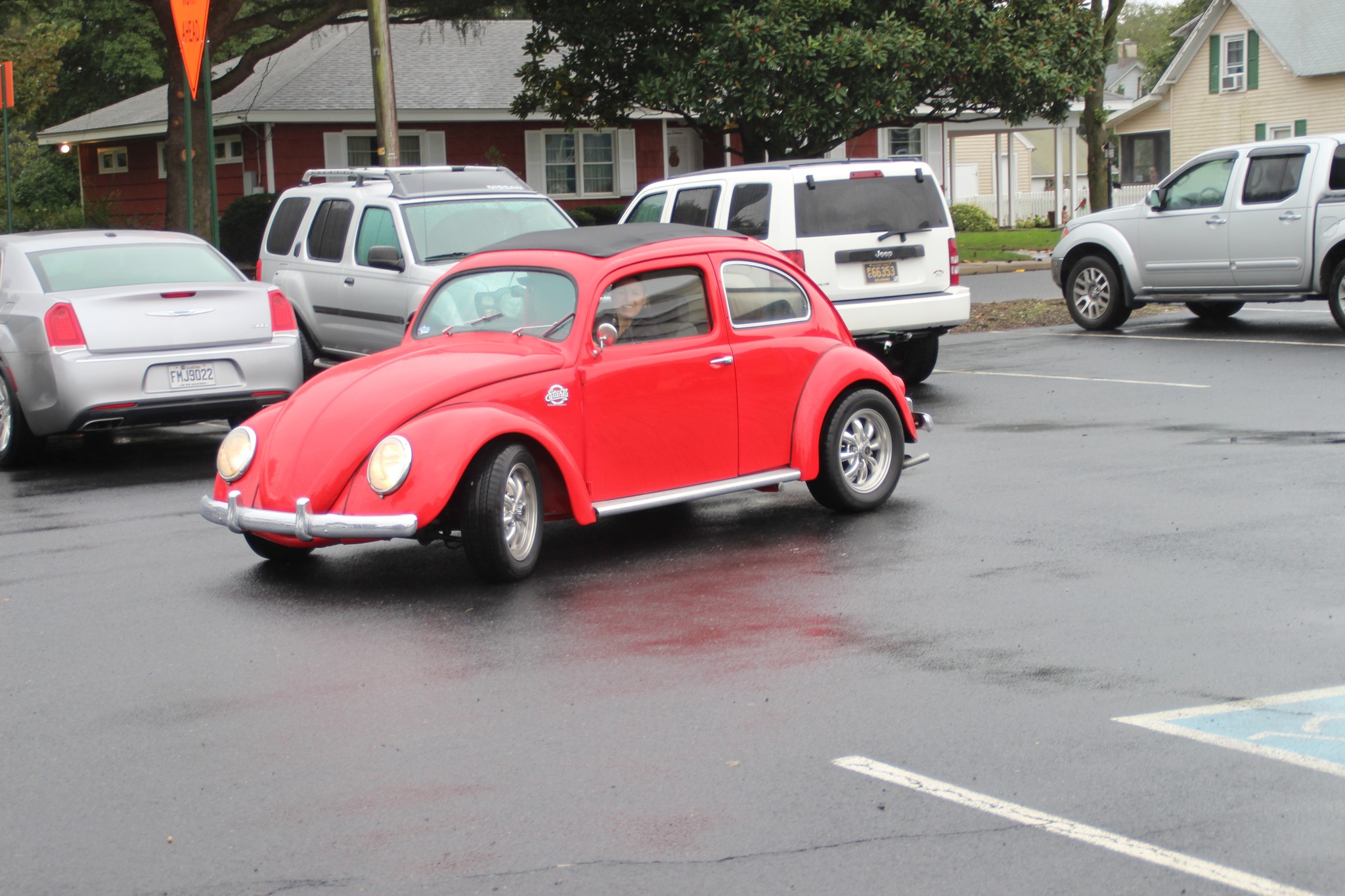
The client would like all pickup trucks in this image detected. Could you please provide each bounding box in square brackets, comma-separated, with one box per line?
[1049, 132, 1345, 332]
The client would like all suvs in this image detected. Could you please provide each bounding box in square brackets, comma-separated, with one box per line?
[259, 165, 583, 367]
[606, 154, 972, 384]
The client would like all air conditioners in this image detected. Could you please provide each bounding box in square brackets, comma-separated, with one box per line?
[1222, 75, 1242, 90]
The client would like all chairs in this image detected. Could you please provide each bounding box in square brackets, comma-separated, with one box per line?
[647, 295, 698, 339]
[1251, 164, 1294, 202]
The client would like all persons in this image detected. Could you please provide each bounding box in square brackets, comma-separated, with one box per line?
[588, 274, 676, 348]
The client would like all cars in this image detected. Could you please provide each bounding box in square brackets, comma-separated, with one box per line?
[200, 223, 939, 584]
[0, 227, 307, 463]
[218, 193, 284, 269]
[660, 438, 673, 446]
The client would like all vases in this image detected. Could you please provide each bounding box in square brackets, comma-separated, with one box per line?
[1048, 212, 1055, 228]
